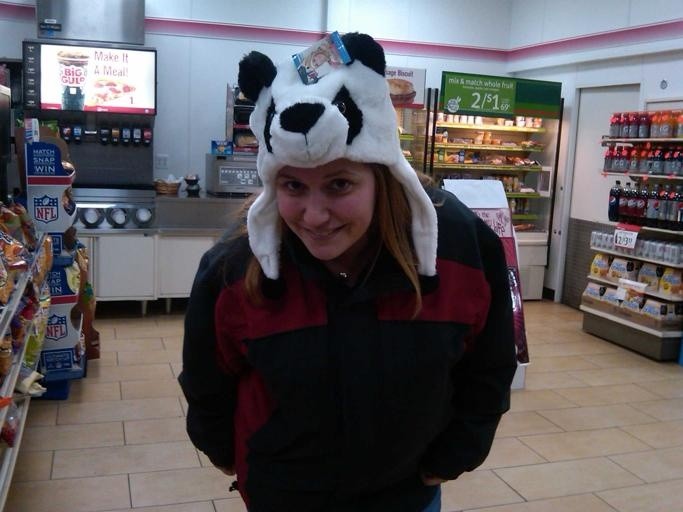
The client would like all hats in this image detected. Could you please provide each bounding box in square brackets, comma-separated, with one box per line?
[237, 31, 441, 282]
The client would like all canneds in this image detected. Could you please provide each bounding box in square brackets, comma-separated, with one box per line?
[587, 230, 683, 269]
[475, 131, 491, 145]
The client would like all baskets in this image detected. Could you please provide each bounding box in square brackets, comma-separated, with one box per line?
[154, 177, 181, 196]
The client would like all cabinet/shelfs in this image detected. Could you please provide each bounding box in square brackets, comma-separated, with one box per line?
[578, 133, 683, 366]
[0, 135, 88, 512]
[392, 87, 564, 301]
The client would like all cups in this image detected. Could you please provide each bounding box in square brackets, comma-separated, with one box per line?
[56, 49, 89, 112]
[83, 208, 153, 225]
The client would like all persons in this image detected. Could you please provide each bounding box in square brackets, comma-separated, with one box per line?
[172, 28, 514, 511]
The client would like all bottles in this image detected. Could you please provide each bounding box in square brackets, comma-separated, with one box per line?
[604, 109, 683, 231]
